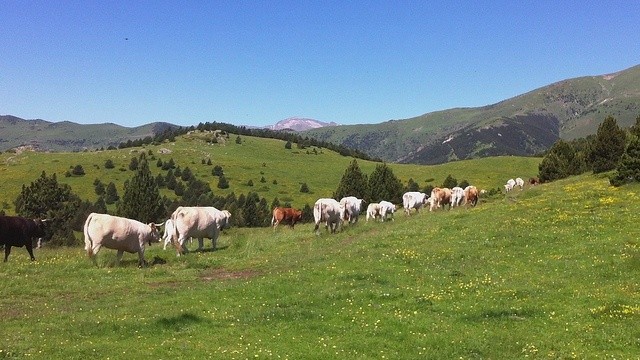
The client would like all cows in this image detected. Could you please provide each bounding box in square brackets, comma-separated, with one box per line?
[337, 196, 365, 230]
[425, 196, 432, 208]
[504, 178, 515, 193]
[270, 206, 305, 231]
[377, 200, 397, 222]
[84, 211, 163, 270]
[366, 202, 380, 226]
[449, 186, 464, 206]
[172, 205, 232, 258]
[163, 218, 193, 258]
[0, 214, 50, 262]
[402, 189, 428, 218]
[429, 186, 453, 216]
[529, 177, 541, 186]
[515, 177, 525, 191]
[460, 186, 481, 207]
[311, 197, 341, 234]
